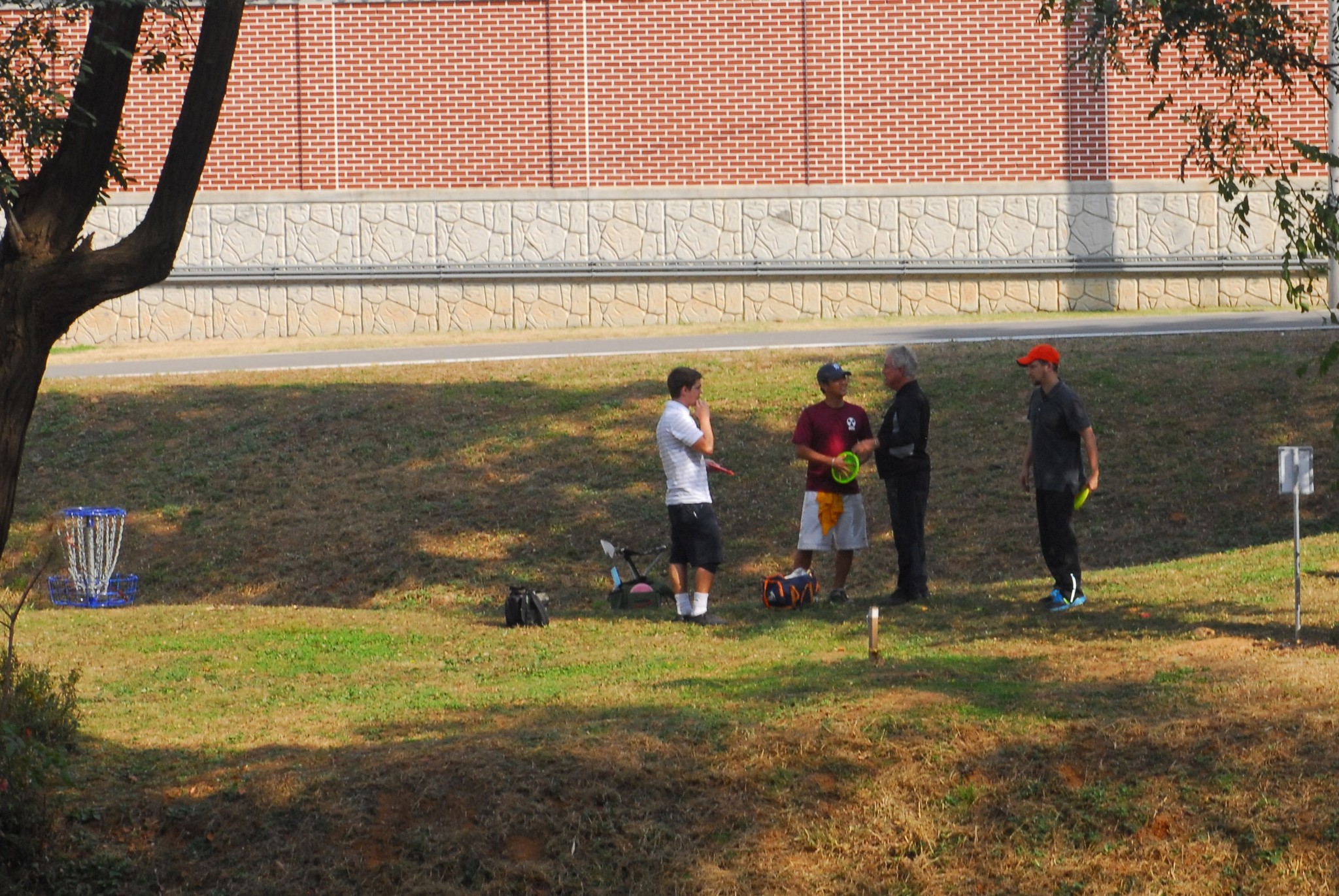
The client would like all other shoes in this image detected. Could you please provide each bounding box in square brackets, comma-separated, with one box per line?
[879, 584, 929, 606]
[675, 614, 690, 623]
[829, 589, 848, 604]
[690, 611, 726, 626]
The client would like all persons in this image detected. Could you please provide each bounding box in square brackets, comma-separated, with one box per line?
[852, 346, 933, 605]
[792, 363, 874, 601]
[1016, 345, 1100, 613]
[656, 366, 727, 625]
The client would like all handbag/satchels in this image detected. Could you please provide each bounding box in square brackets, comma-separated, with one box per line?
[762, 567, 820, 611]
[504, 586, 550, 628]
[609, 578, 661, 609]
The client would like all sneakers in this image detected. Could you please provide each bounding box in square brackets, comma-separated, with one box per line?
[1040, 589, 1061, 602]
[1046, 594, 1087, 612]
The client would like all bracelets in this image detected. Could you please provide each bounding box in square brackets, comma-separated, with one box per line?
[831, 458, 834, 467]
[874, 438, 878, 449]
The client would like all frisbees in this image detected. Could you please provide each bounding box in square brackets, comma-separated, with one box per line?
[831, 451, 860, 484]
[1074, 484, 1090, 510]
[706, 461, 735, 476]
[630, 583, 654, 592]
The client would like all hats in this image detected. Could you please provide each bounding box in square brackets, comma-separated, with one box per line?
[1016, 344, 1061, 366]
[817, 362, 852, 383]
